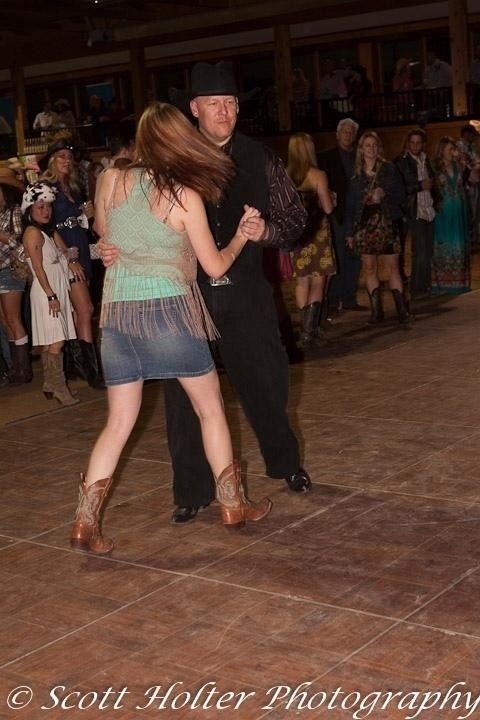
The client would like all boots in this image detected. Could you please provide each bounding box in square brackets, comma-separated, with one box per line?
[2, 338, 106, 406]
[211, 459, 272, 531]
[70, 471, 113, 554]
[298, 287, 417, 348]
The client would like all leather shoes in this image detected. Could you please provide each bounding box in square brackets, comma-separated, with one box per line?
[285, 469, 311, 494]
[170, 502, 210, 524]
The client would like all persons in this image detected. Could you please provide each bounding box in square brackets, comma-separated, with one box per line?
[69, 99, 273, 558]
[97, 65, 313, 524]
[1, 47, 480, 406]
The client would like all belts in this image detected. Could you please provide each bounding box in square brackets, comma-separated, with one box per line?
[202, 276, 235, 286]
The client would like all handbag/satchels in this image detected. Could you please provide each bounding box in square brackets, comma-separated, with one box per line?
[8, 246, 31, 281]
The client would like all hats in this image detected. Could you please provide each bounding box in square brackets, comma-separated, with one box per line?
[191, 64, 240, 98]
[0, 138, 81, 214]
